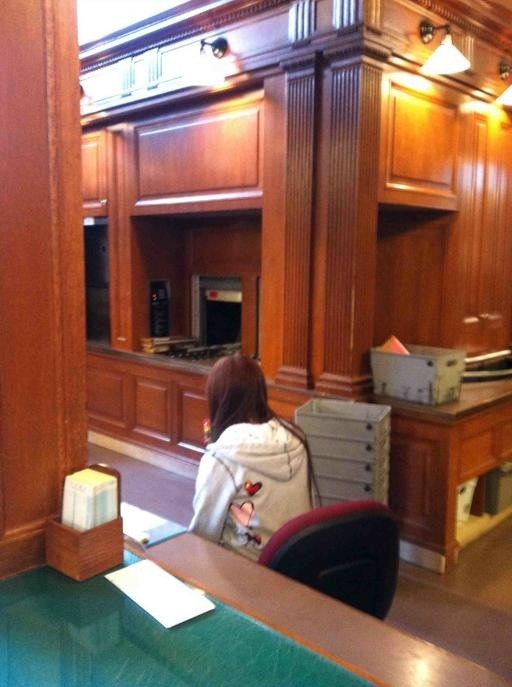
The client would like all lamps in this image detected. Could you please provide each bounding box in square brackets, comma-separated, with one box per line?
[189, 38, 227, 81]
[419, 18, 472, 77]
[495, 65, 512, 109]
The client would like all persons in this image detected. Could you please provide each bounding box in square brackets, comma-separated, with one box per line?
[189, 355, 322, 563]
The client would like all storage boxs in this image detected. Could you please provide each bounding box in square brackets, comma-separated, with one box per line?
[371, 344, 467, 406]
[294, 400, 390, 505]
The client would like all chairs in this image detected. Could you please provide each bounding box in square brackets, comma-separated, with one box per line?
[258, 501, 400, 622]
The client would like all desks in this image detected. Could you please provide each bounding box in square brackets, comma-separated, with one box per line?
[1, 502, 511, 686]
[368, 378, 511, 568]
